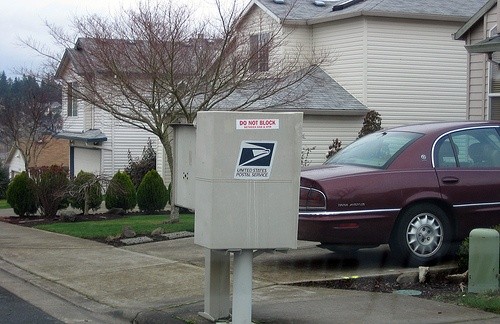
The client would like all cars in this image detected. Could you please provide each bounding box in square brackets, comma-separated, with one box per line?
[300, 121, 500, 266]
